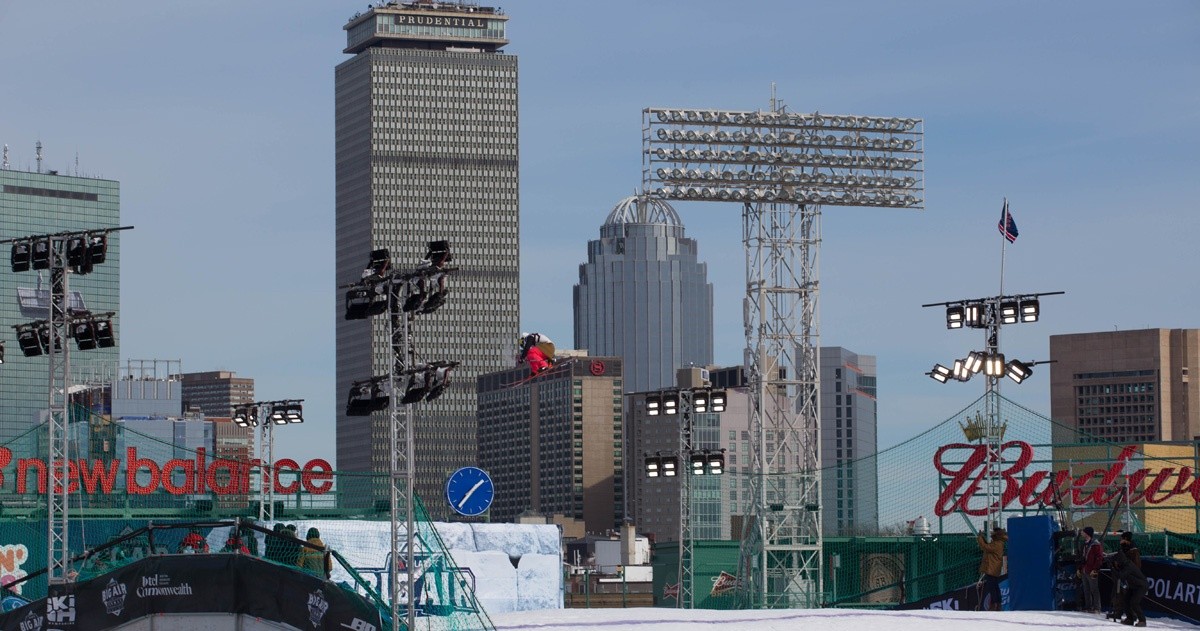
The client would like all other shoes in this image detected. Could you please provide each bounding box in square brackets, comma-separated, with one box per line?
[1121, 619, 1134, 625]
[1135, 621, 1146, 627]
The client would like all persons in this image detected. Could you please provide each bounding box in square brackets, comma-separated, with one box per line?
[1105, 531, 1149, 627]
[228, 518, 259, 557]
[264, 523, 303, 567]
[296, 527, 332, 580]
[976, 527, 1005, 611]
[178, 526, 210, 553]
[1076, 527, 1103, 614]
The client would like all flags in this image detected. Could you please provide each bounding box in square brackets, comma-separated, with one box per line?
[997, 197, 1019, 243]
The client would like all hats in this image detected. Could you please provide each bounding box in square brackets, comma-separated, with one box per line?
[1083, 527, 1094, 538]
[1121, 532, 1132, 542]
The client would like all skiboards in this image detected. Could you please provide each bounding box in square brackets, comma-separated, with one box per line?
[499, 355, 576, 392]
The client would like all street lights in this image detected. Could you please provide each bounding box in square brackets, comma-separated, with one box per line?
[922, 289, 1068, 610]
[344, 235, 460, 630]
[622, 384, 750, 611]
[0, 224, 137, 589]
[229, 397, 305, 531]
